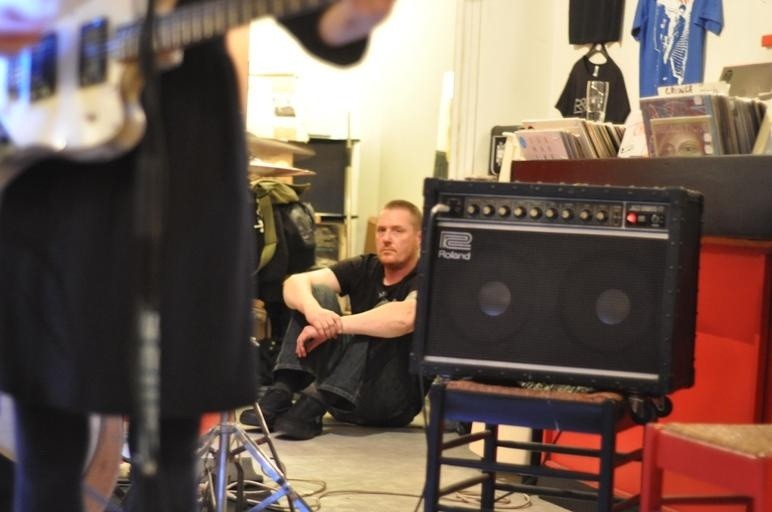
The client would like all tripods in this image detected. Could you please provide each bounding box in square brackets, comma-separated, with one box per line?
[193, 410, 314, 512]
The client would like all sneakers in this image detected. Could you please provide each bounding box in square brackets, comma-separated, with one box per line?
[240, 388, 292, 427]
[276, 394, 327, 439]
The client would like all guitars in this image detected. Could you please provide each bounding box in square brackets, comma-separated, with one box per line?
[0, 0, 343, 186]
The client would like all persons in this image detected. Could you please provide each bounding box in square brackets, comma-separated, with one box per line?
[237, 198, 430, 442]
[1, 1, 398, 511]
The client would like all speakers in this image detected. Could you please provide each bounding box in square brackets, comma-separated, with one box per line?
[408, 177, 703, 393]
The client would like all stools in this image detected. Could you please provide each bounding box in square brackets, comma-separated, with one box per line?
[418, 366, 676, 510]
[632, 414, 772, 511]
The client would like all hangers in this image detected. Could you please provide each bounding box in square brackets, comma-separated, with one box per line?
[578, 39, 611, 67]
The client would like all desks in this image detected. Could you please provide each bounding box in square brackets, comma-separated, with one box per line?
[501, 148, 772, 512]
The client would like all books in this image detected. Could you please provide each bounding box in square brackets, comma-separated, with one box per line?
[511, 80, 772, 162]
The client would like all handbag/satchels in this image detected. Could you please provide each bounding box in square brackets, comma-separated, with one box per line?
[246, 180, 315, 281]
